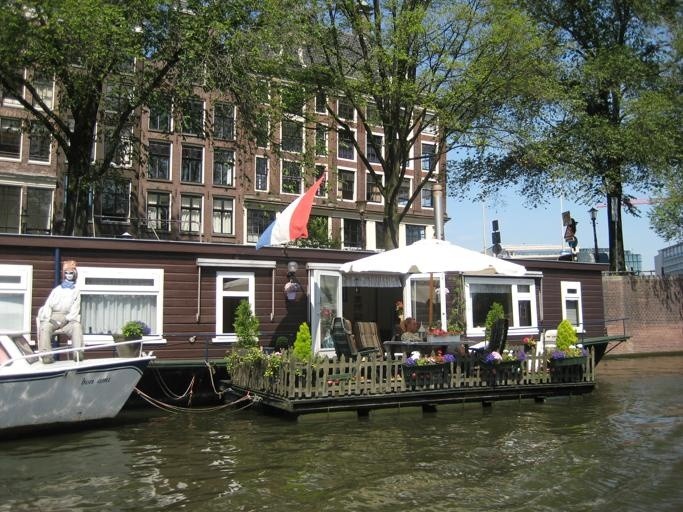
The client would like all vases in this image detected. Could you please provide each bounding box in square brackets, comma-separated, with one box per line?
[286, 291, 298, 300]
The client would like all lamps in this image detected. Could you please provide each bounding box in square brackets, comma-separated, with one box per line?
[284, 261, 300, 278]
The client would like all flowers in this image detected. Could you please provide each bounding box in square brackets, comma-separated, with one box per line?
[283, 281, 299, 293]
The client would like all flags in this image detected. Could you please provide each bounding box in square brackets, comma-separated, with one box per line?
[255, 174, 324, 253]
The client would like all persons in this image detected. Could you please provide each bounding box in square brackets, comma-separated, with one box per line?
[36, 259, 82, 365]
[401, 317, 424, 342]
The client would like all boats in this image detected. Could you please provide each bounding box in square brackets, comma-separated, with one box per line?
[0, 336, 166, 431]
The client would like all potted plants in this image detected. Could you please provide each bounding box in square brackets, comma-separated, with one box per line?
[124, 322, 144, 348]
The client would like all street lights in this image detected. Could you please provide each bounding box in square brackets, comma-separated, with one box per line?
[355, 200, 371, 251]
[589, 207, 599, 263]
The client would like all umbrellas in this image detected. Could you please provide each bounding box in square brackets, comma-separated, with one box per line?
[340, 234, 528, 330]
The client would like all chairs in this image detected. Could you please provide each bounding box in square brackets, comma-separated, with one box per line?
[330, 318, 510, 381]
